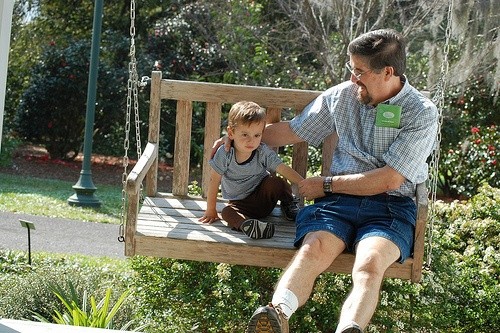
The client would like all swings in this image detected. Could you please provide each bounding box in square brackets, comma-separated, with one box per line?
[117, 0, 454, 284]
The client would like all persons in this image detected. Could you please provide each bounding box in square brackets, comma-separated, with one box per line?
[198, 101, 305, 240]
[211, 29, 441, 333]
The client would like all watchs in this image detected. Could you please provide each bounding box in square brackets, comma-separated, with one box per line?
[322, 176, 333, 195]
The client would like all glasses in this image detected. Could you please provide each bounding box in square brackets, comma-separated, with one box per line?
[346, 60, 376, 81]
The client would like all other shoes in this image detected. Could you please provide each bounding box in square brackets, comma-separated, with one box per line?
[335, 321, 364, 333]
[280, 196, 302, 221]
[240, 218, 276, 239]
[247, 302, 289, 333]
